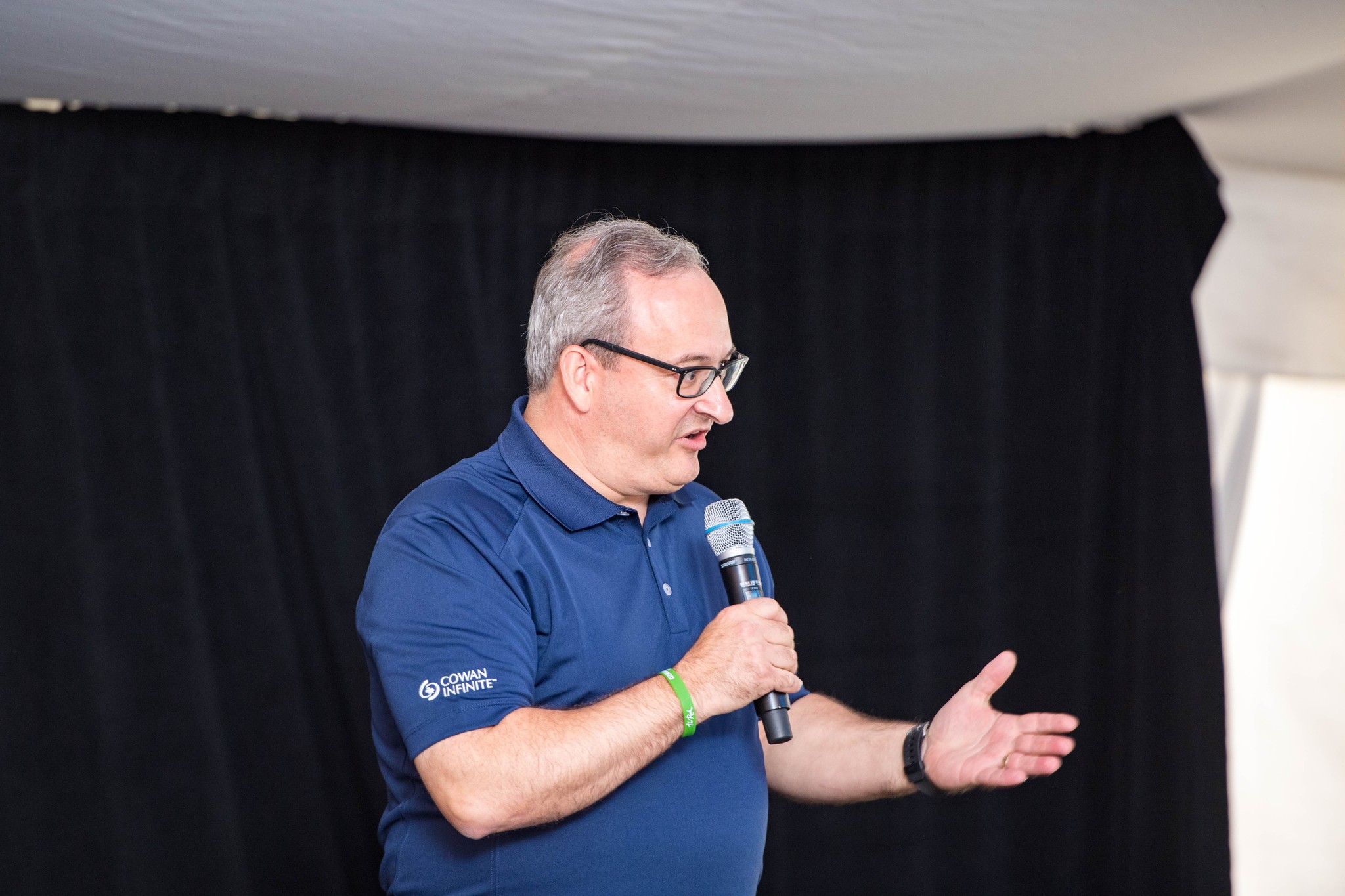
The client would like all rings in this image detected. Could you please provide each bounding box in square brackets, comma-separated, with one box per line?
[1002, 752, 1015, 768]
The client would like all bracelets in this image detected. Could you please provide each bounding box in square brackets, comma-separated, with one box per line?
[903, 720, 965, 796]
[662, 667, 696, 737]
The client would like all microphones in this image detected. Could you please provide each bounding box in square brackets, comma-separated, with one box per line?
[703, 498, 793, 745]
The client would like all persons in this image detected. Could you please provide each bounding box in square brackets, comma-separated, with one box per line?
[355, 219, 1078, 896]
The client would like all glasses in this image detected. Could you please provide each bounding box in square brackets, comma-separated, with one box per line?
[580, 339, 750, 399]
